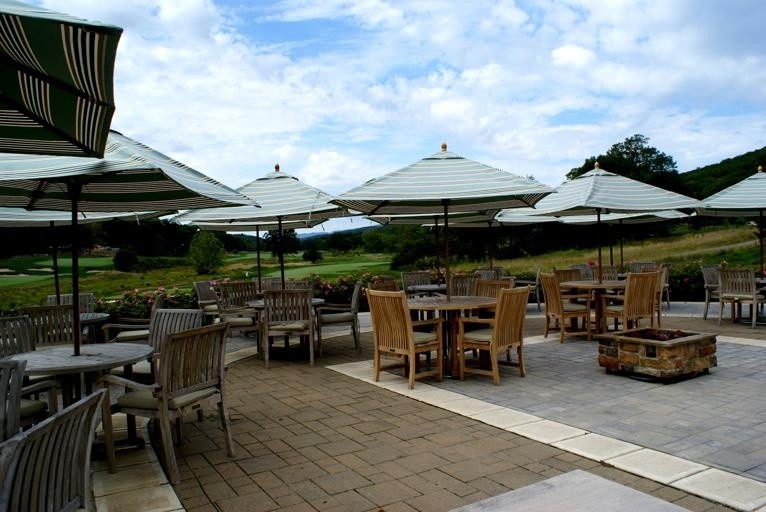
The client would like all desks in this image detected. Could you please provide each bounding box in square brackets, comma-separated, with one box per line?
[404, 293, 499, 379]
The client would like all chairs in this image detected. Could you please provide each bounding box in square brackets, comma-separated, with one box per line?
[1, 384, 109, 512]
[698, 263, 765, 328]
[371, 263, 516, 342]
[116, 293, 167, 348]
[105, 322, 237, 484]
[125, 309, 207, 444]
[314, 280, 362, 361]
[196, 281, 225, 315]
[539, 264, 675, 345]
[214, 281, 261, 339]
[364, 287, 446, 389]
[456, 283, 534, 384]
[1, 289, 121, 476]
[256, 287, 317, 369]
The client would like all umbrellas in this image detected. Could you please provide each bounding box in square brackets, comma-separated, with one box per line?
[696, 166, 766, 280]
[169, 162, 352, 302]
[500, 162, 712, 284]
[0, 0, 123, 160]
[0, 129, 262, 356]
[328, 143, 557, 302]
